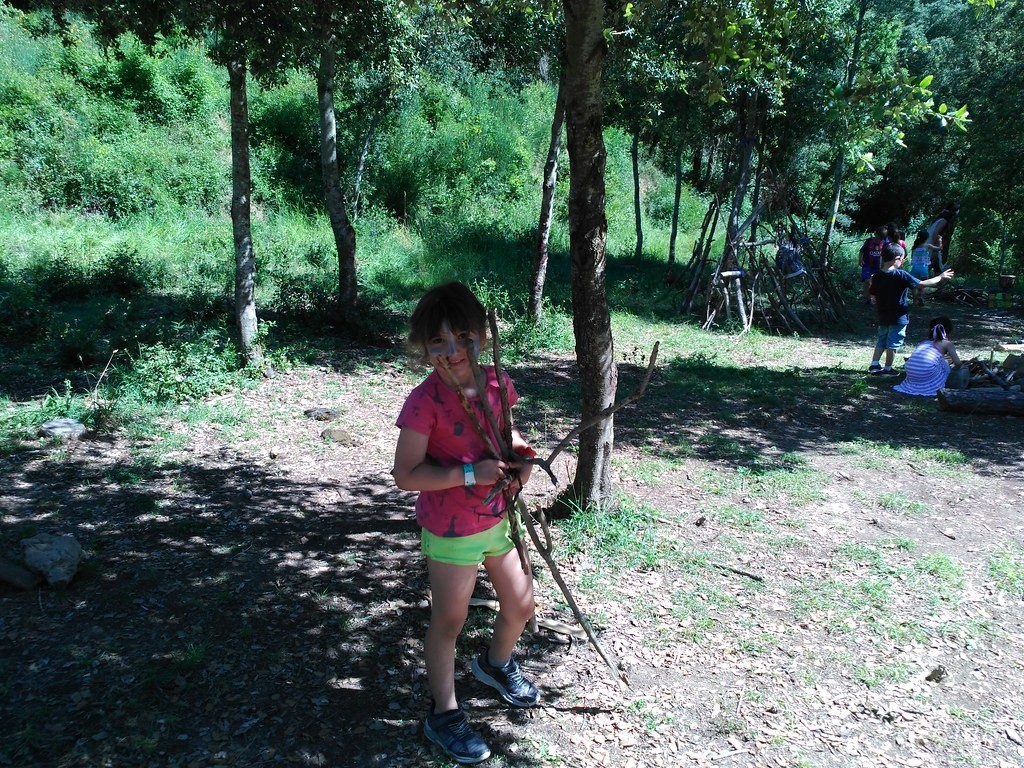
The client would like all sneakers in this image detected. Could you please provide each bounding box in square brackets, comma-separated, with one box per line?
[869, 364, 883, 373]
[470, 647, 541, 706]
[423, 706, 491, 763]
[881, 367, 900, 377]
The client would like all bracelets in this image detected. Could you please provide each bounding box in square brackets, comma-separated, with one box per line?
[463, 463, 475, 487]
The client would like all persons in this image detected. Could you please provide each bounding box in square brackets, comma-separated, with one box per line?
[857, 211, 964, 397]
[393, 280, 542, 764]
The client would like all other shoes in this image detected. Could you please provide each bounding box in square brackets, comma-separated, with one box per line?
[914, 294, 925, 308]
[860, 298, 877, 305]
